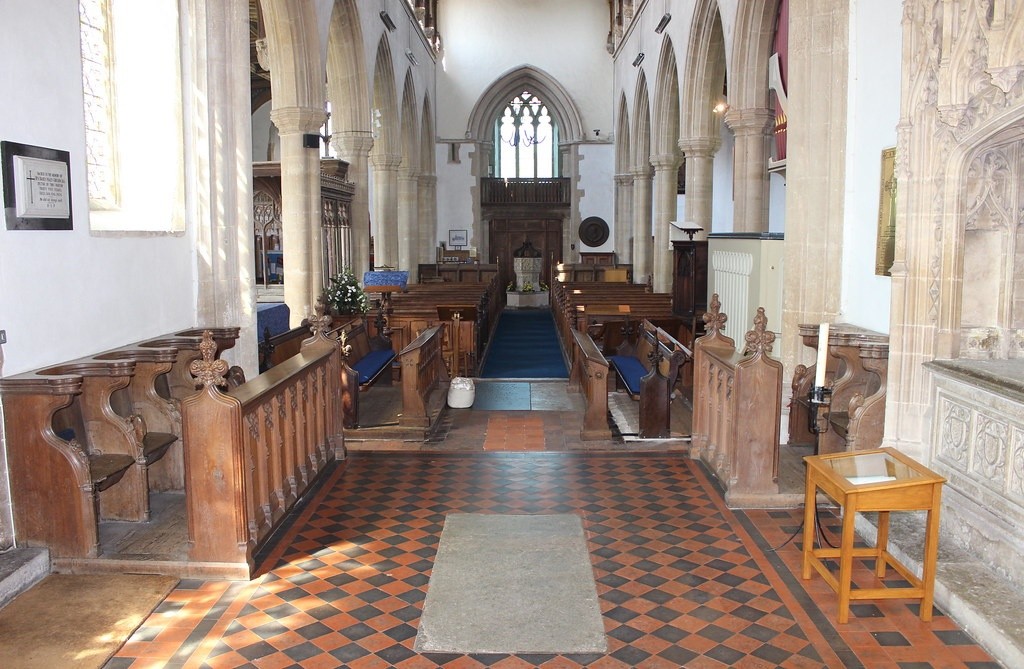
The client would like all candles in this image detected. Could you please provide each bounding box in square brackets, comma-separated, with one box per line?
[813, 323, 830, 401]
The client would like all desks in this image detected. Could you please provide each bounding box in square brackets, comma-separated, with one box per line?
[257, 302, 291, 341]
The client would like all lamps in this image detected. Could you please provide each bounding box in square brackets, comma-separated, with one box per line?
[380, 10, 398, 33]
[632, 53, 645, 69]
[654, 12, 671, 34]
[405, 47, 420, 67]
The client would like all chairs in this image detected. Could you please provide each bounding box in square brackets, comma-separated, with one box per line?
[786, 321, 866, 453]
[174, 326, 247, 394]
[79, 345, 184, 492]
[819, 341, 890, 452]
[0, 362, 150, 556]
[125, 331, 203, 416]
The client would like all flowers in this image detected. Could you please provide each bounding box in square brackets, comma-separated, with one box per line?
[321, 263, 369, 314]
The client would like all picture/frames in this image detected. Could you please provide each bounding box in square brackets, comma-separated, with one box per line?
[455, 246, 461, 251]
[449, 229, 468, 246]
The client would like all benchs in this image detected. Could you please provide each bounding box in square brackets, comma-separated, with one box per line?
[258, 278, 695, 445]
